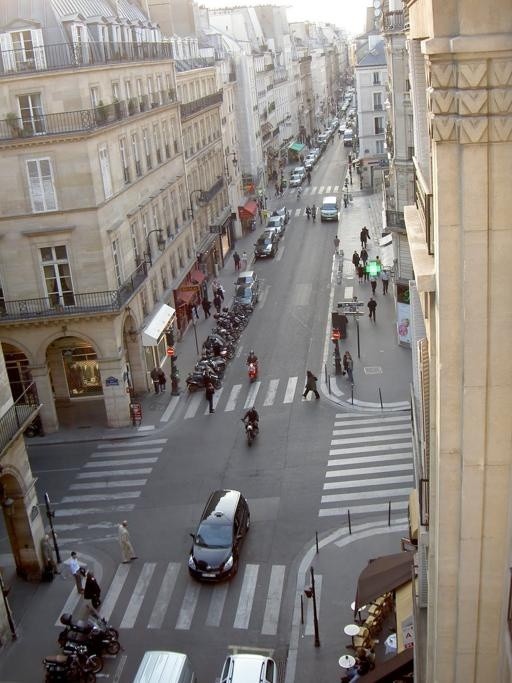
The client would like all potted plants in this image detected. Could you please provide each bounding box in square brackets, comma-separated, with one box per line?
[94, 99, 108, 127]
[20, 122, 35, 137]
[4, 110, 23, 137]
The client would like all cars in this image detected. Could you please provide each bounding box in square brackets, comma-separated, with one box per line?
[217, 652, 278, 682]
[232, 270, 259, 291]
[233, 281, 259, 314]
[255, 202, 292, 258]
[289, 87, 356, 188]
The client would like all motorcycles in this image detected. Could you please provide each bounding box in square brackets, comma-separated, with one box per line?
[240, 418, 258, 446]
[40, 604, 122, 682]
[184, 305, 249, 393]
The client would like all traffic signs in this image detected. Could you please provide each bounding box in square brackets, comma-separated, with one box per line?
[338, 301, 365, 307]
[344, 312, 365, 316]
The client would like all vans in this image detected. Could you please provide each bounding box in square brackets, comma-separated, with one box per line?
[131, 648, 199, 682]
[185, 486, 253, 585]
[321, 196, 338, 222]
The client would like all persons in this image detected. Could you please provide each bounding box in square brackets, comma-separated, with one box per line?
[63, 552, 85, 593]
[43, 534, 62, 575]
[84, 571, 101, 609]
[118, 520, 139, 563]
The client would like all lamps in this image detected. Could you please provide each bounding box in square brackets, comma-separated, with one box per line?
[186, 186, 210, 222]
[0, 463, 18, 519]
[223, 148, 237, 177]
[125, 305, 139, 343]
[147, 225, 168, 255]
[61, 324, 75, 366]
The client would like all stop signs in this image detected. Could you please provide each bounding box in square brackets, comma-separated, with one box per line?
[167, 347, 174, 355]
[331, 329, 340, 339]
